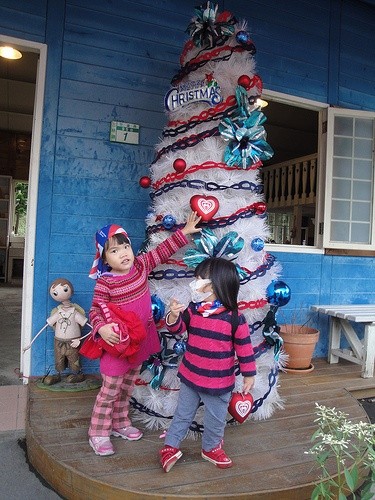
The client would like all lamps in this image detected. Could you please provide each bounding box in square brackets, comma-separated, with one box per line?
[0, 45, 22, 60]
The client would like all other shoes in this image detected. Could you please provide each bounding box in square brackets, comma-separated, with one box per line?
[111, 426, 143, 441]
[88, 437, 115, 456]
[159, 448, 183, 473]
[201, 444, 232, 469]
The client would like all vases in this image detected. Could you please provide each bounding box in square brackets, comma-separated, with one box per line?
[275, 323, 319, 375]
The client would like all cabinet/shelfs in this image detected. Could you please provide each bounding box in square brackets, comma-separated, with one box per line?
[0, 175, 24, 283]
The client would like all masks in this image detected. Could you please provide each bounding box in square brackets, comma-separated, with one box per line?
[189, 279, 214, 303]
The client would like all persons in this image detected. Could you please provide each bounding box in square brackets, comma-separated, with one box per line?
[87, 211, 203, 456]
[159, 257, 256, 474]
[21, 277, 97, 386]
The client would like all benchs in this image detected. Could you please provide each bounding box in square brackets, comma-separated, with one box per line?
[310, 304, 375, 378]
